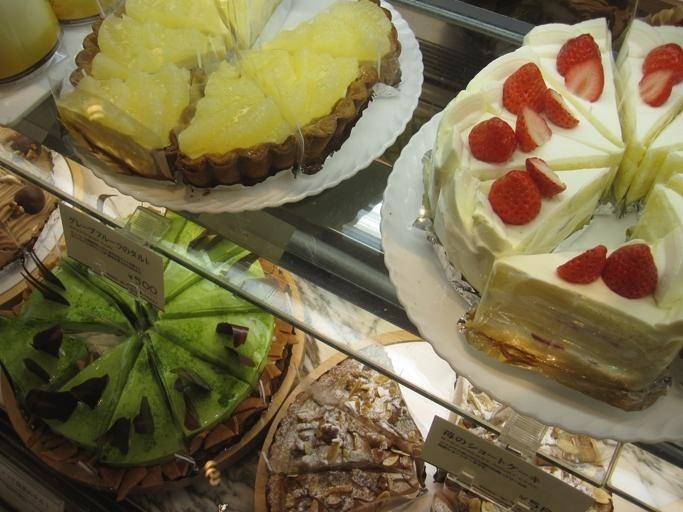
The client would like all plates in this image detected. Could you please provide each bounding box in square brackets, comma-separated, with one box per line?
[57, 1, 425, 217]
[377, 95, 683, 447]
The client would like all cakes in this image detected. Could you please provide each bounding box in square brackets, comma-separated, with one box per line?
[267, 345, 612, 512]
[65, 0, 402, 191]
[0, 126, 53, 270]
[426, 18, 683, 409]
[1, 209, 277, 467]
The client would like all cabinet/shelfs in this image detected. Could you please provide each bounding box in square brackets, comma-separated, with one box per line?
[1, 0, 682, 512]
